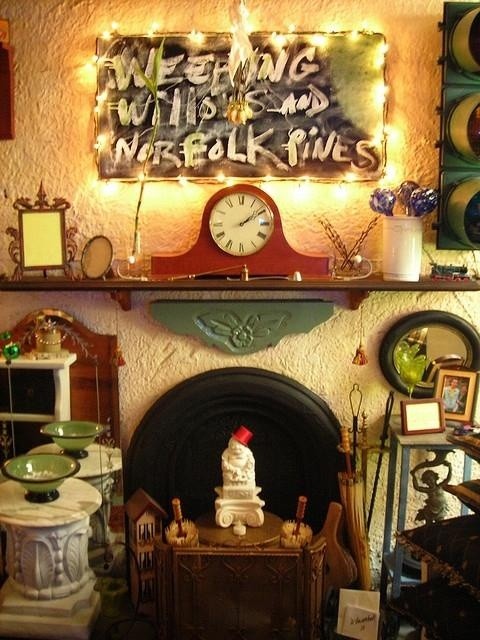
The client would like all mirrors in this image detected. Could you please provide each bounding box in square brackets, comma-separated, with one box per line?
[6, 178, 77, 282]
[378, 311, 480, 399]
[399, 397, 445, 436]
[81, 235, 113, 282]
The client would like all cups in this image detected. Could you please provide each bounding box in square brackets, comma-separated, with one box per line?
[381, 215, 423, 281]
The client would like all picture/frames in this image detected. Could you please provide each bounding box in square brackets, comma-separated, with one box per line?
[431, 365, 480, 429]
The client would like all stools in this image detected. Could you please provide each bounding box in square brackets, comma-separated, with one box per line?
[380, 414, 473, 607]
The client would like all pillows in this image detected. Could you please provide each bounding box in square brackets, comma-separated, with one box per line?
[442, 479, 480, 513]
[394, 513, 479, 604]
[446, 433, 480, 465]
[386, 577, 480, 640]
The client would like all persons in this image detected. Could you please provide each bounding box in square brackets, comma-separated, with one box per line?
[456, 384, 467, 411]
[441, 378, 460, 413]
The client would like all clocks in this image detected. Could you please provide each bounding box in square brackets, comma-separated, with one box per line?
[153, 184, 330, 279]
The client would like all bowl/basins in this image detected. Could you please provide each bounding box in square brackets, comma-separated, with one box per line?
[40, 421, 104, 459]
[3, 453, 80, 504]
